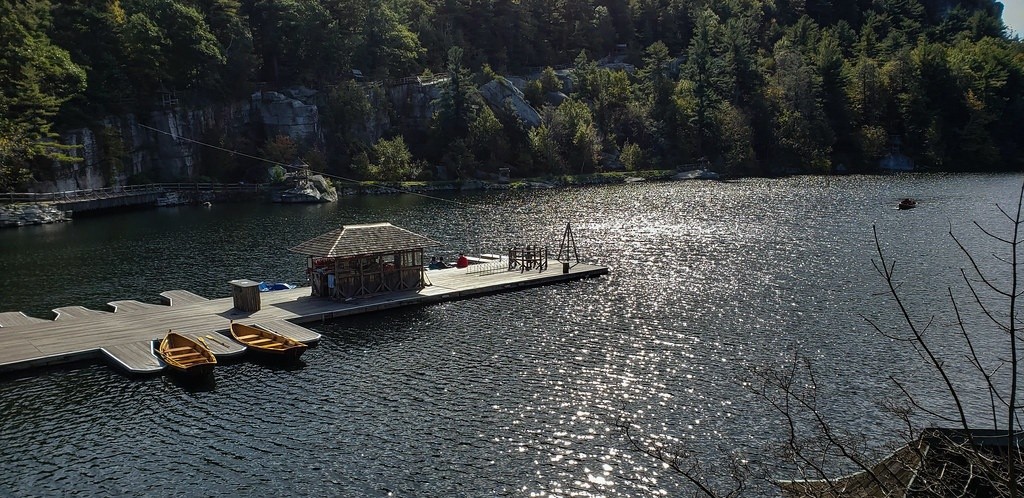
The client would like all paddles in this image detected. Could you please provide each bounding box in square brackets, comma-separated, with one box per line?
[198, 337, 210, 350]
[205, 334, 230, 347]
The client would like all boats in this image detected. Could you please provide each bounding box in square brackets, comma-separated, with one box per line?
[229, 319, 308, 364]
[899, 197, 919, 210]
[153, 328, 218, 379]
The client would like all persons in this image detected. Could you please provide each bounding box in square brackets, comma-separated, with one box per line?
[429, 256, 452, 268]
[454, 253, 468, 269]
